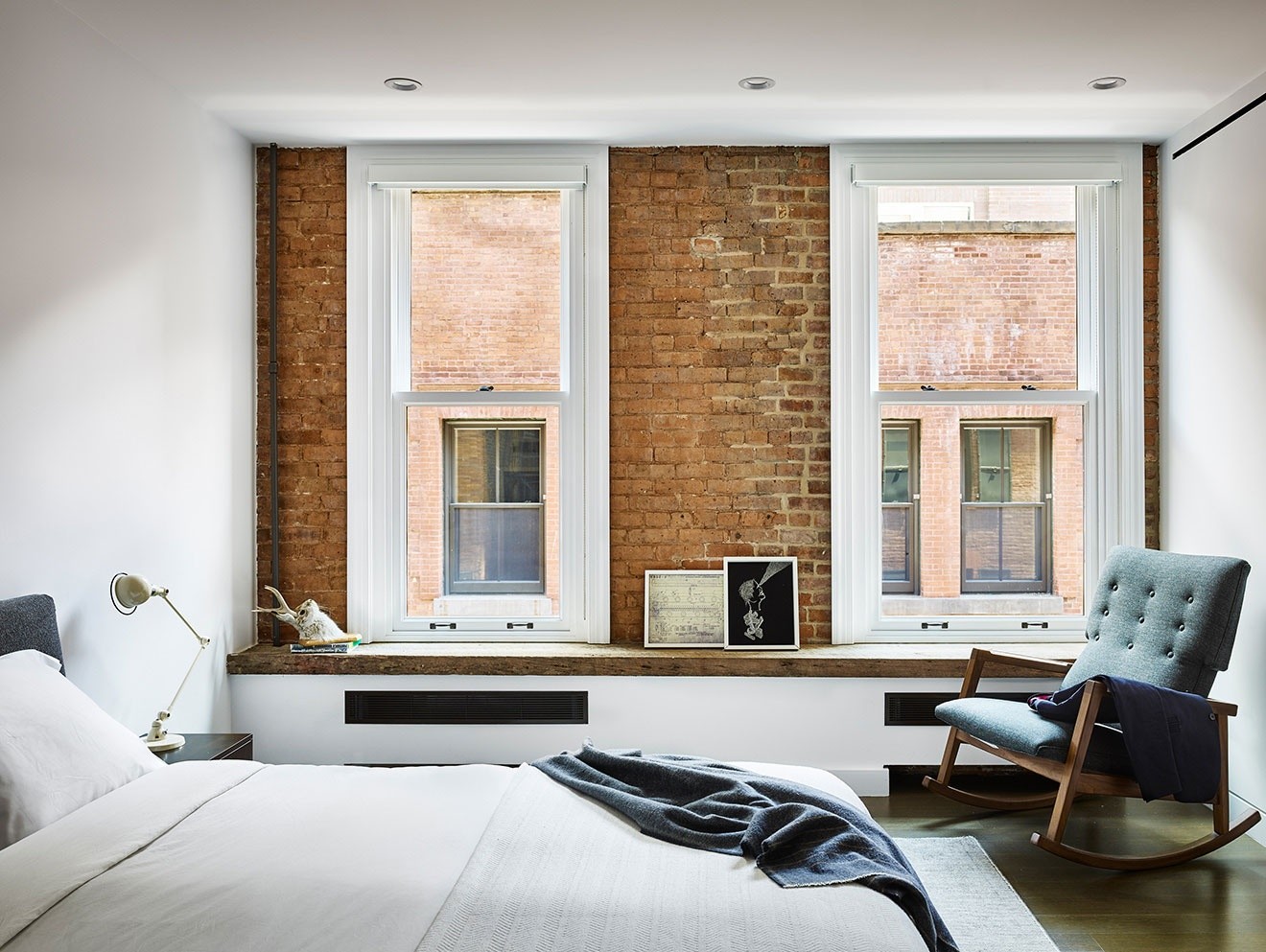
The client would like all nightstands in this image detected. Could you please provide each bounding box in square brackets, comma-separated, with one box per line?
[139, 733, 254, 761]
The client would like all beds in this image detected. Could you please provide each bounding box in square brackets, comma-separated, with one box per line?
[0, 594, 931, 952]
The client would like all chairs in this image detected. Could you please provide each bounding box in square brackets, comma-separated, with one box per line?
[922, 546, 1261, 870]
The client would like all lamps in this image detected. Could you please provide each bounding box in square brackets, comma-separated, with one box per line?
[110, 572, 210, 751]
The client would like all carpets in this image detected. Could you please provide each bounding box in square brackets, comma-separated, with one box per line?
[890, 835, 1061, 952]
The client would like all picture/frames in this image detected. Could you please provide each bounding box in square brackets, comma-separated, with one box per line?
[644, 569, 723, 648]
[723, 556, 800, 650]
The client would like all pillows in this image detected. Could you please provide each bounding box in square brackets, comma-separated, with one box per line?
[0, 649, 168, 850]
